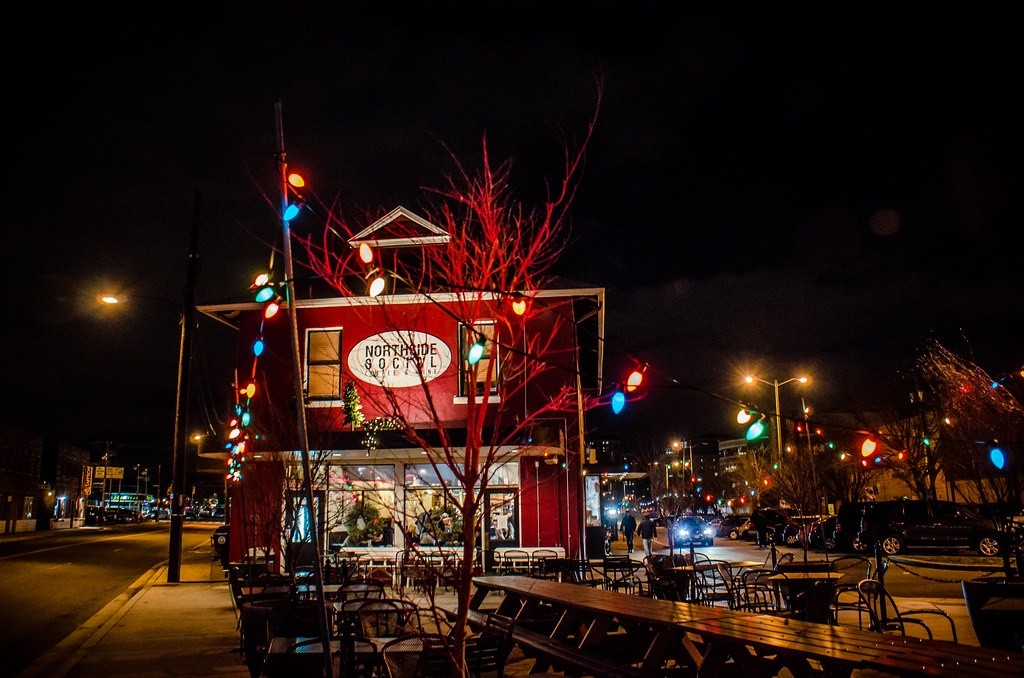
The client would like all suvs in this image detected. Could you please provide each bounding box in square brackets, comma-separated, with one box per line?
[833, 498, 1021, 557]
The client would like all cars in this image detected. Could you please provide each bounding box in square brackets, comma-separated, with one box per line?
[668, 516, 713, 547]
[644, 511, 833, 551]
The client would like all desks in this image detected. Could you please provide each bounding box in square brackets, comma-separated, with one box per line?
[448, 575, 1024, 678]
[333, 596, 502, 612]
[268, 637, 475, 656]
[769, 572, 846, 580]
[241, 585, 380, 596]
[668, 561, 764, 570]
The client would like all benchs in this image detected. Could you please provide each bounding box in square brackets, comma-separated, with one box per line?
[403, 547, 480, 588]
[435, 603, 649, 678]
[330, 547, 405, 588]
[491, 546, 566, 583]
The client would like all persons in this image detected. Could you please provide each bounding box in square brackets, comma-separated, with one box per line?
[342, 535, 353, 546]
[373, 532, 384, 547]
[637, 514, 657, 556]
[620, 510, 637, 553]
[755, 511, 768, 547]
[53, 499, 64, 522]
[408, 509, 445, 544]
[474, 527, 481, 557]
[490, 521, 514, 539]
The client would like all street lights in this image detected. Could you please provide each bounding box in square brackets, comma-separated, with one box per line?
[744, 376, 808, 499]
[98, 294, 191, 584]
[654, 462, 669, 496]
[673, 443, 708, 490]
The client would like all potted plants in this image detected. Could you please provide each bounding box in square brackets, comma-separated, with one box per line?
[230, 420, 351, 678]
[733, 444, 834, 622]
[916, 338, 1023, 650]
[645, 442, 689, 601]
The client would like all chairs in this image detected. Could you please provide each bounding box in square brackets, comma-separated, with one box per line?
[228, 550, 957, 678]
